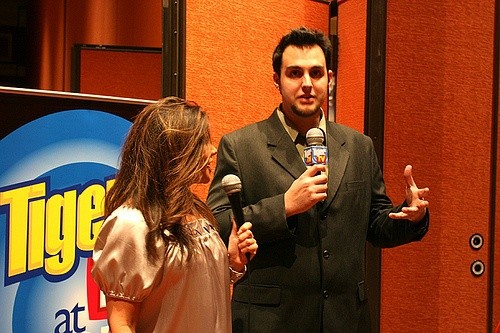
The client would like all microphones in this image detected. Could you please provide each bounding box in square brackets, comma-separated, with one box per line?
[302, 128, 329, 207]
[221, 174, 252, 262]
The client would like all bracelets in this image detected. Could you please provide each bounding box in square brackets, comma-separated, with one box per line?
[226, 265, 247, 284]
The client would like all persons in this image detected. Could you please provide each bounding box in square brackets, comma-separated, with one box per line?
[203, 26, 431, 333]
[91, 95, 259, 333]
